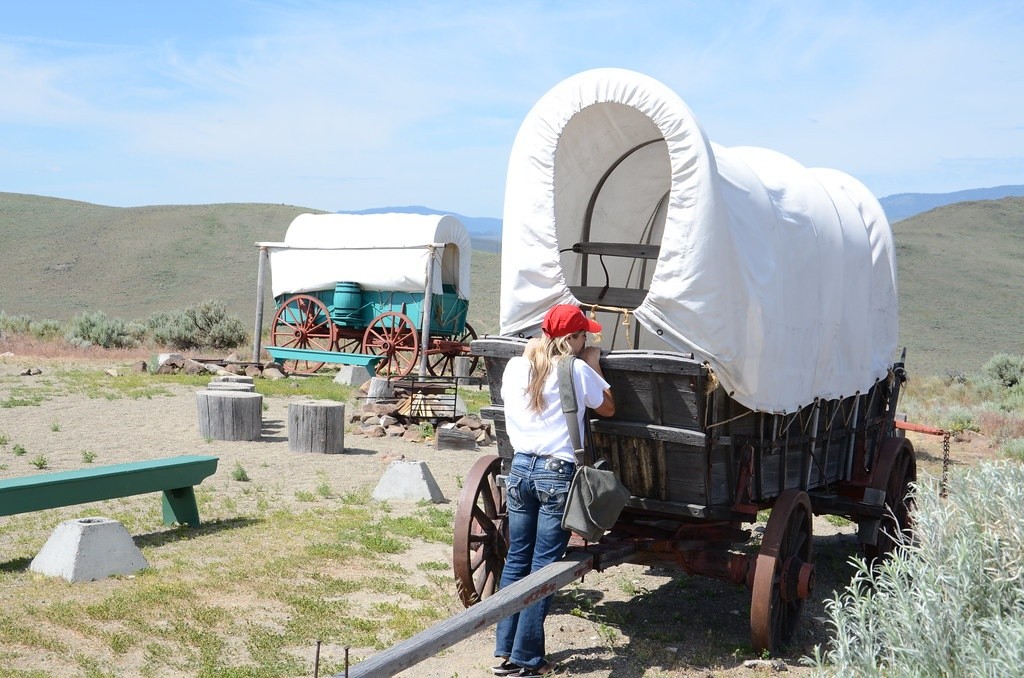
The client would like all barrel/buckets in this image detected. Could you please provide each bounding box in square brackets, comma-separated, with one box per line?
[333, 281, 362, 318]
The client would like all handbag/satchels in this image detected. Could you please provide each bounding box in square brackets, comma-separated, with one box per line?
[561, 465, 632, 544]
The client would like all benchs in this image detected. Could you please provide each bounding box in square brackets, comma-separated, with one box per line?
[263, 346, 388, 379]
[1, 453, 222, 529]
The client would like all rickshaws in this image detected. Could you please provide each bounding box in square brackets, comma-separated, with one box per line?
[267, 212, 480, 381]
[312, 68, 918, 678]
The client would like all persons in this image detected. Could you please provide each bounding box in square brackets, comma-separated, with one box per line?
[492, 303, 614, 678]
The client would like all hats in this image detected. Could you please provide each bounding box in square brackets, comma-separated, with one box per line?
[542, 304, 602, 341]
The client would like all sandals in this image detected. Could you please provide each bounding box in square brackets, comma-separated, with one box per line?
[492, 660, 524, 675]
[506, 659, 560, 678]
